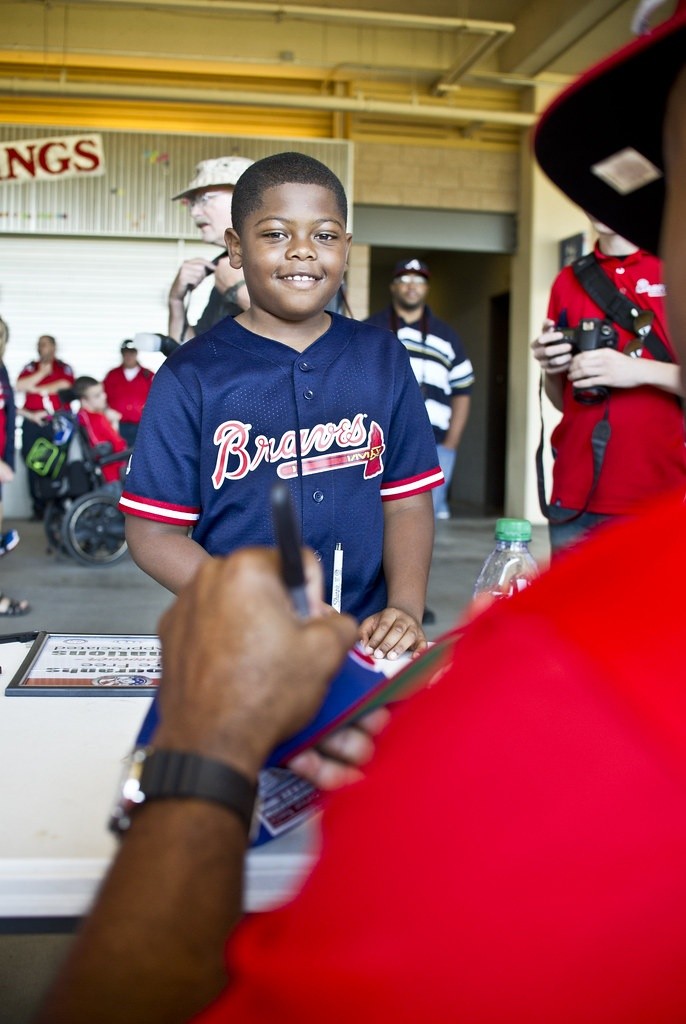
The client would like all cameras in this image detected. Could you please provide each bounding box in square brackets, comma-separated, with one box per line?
[551, 316, 618, 405]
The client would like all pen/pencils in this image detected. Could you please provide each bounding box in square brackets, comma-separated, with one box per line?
[331, 543, 344, 615]
[270, 482, 310, 620]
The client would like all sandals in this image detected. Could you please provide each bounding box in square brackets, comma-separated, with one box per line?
[0, 594, 29, 615]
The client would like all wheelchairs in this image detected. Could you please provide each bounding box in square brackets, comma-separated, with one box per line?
[41, 408, 133, 567]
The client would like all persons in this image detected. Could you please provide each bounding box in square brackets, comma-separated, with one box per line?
[115, 153, 445, 658]
[37, 0, 686, 1024]
[359, 258, 475, 626]
[0, 318, 155, 618]
[168, 156, 340, 348]
[531, 208, 686, 566]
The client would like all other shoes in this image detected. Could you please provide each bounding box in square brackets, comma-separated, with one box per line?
[0, 530, 21, 555]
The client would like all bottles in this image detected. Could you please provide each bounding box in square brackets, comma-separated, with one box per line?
[472, 518, 538, 617]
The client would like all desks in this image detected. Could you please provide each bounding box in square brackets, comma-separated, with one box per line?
[0, 629, 444, 935]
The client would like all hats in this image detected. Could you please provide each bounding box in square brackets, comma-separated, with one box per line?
[534, 0, 686, 263]
[169, 157, 258, 201]
[120, 339, 138, 351]
[390, 255, 430, 283]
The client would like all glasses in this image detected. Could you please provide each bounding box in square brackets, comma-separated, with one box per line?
[187, 192, 235, 206]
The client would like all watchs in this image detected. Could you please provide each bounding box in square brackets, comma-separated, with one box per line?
[110, 746, 262, 844]
